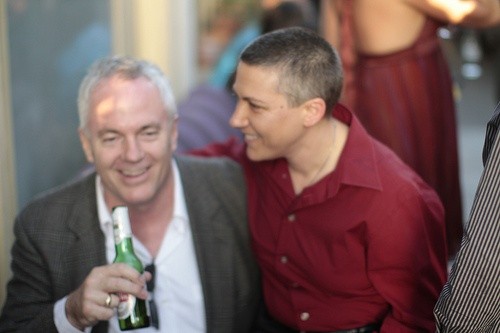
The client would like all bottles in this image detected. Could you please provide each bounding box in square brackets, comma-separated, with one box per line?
[112, 205, 151, 330]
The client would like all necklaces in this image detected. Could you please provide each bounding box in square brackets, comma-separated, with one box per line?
[303, 120, 337, 186]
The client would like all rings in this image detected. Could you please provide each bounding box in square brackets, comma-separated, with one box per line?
[105, 293, 112, 308]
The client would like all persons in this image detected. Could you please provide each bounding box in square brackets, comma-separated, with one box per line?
[183, 25, 448, 333]
[318, 0, 500, 261]
[433, 101, 500, 333]
[172, 0, 317, 153]
[0, 54, 265, 333]
